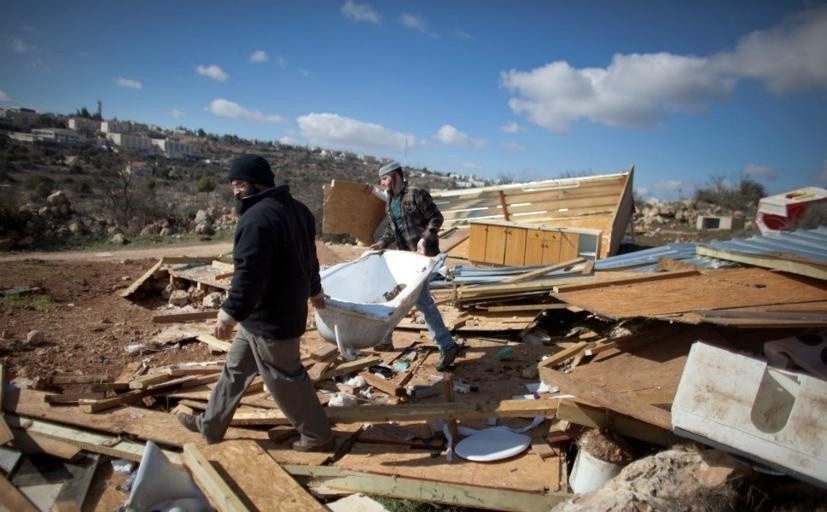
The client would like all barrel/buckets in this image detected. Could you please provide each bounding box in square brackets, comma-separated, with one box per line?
[568, 447, 620, 497]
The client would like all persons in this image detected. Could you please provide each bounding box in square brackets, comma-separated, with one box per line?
[368, 161, 462, 372]
[178, 153, 336, 450]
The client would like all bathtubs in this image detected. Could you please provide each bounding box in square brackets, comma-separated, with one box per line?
[312, 247, 448, 349]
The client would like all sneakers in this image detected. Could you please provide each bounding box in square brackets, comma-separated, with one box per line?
[374, 342, 394, 352]
[435, 343, 461, 371]
[179, 412, 201, 433]
[287, 436, 336, 451]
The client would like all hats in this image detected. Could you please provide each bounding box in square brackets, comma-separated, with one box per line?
[229, 153, 275, 186]
[378, 162, 400, 177]
